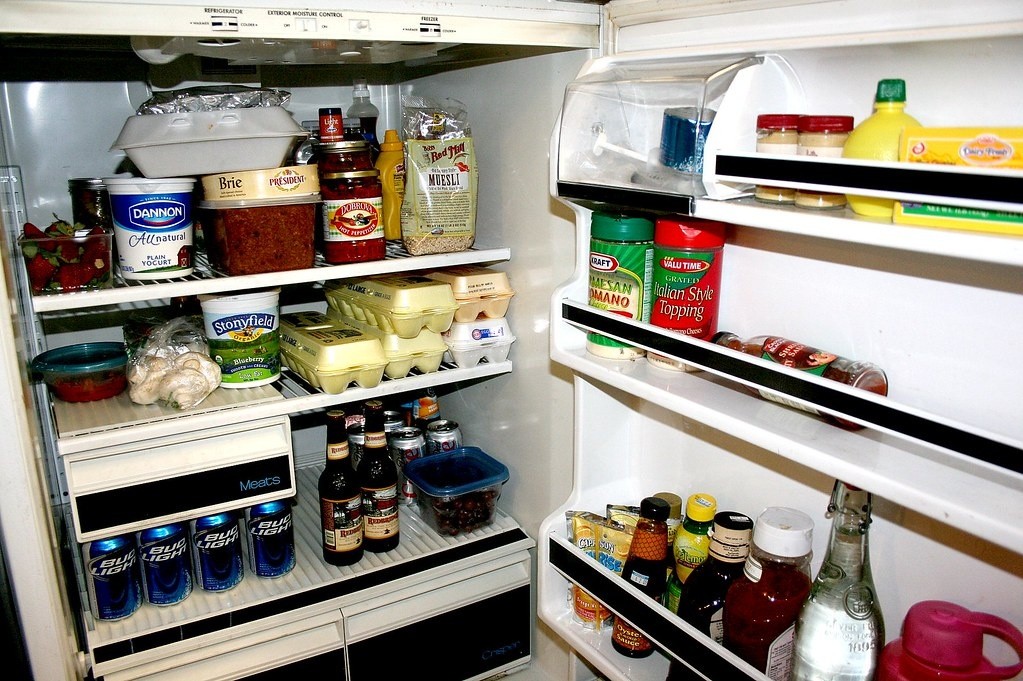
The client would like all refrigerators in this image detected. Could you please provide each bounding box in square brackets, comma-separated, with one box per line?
[0, 0, 1021, 679]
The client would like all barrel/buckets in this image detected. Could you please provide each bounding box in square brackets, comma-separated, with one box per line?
[101, 176, 196, 279]
[197, 288, 282, 388]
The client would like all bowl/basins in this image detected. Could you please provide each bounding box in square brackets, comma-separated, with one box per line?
[278, 267, 516, 393]
[404, 446, 510, 537]
[32, 342, 131, 403]
[110, 107, 310, 178]
[19, 229, 113, 292]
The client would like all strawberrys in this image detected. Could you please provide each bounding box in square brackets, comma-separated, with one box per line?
[20, 211, 110, 292]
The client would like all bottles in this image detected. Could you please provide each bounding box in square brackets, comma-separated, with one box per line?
[661, 494, 717, 615]
[757, 80, 922, 217]
[665, 511, 754, 681]
[721, 507, 815, 681]
[297, 80, 405, 241]
[319, 410, 362, 566]
[787, 482, 886, 681]
[612, 493, 682, 658]
[358, 401, 396, 552]
[713, 331, 889, 428]
[875, 601, 1023, 681]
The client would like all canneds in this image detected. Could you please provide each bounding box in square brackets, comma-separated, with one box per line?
[754, 114, 855, 210]
[347, 410, 464, 507]
[306, 139, 387, 264]
[585, 210, 723, 371]
[90, 499, 297, 622]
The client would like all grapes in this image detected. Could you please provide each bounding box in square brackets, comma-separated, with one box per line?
[433, 492, 492, 535]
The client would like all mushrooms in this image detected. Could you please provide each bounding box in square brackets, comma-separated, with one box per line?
[126, 351, 221, 409]
